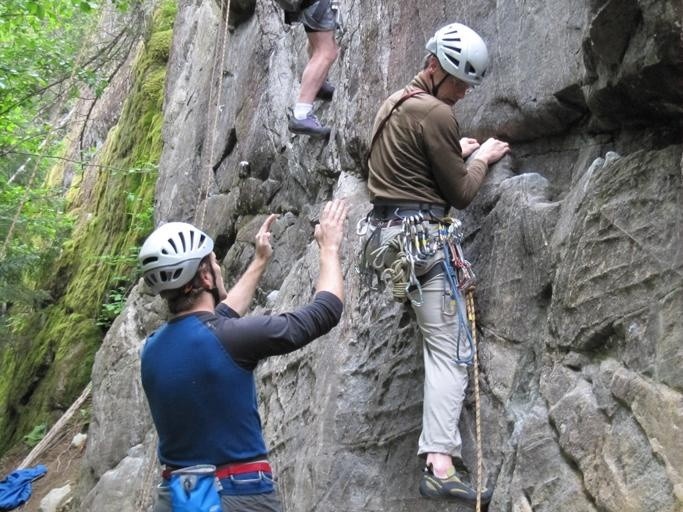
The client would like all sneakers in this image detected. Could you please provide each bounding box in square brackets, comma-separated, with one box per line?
[418, 466, 493, 500]
[287, 113, 331, 137]
[316, 83, 336, 102]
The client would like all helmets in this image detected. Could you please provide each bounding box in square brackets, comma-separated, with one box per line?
[137, 220, 215, 295]
[425, 22, 490, 86]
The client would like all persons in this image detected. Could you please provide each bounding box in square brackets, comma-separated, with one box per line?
[276, 0, 337, 136]
[141, 199, 348, 512]
[360, 23, 511, 505]
